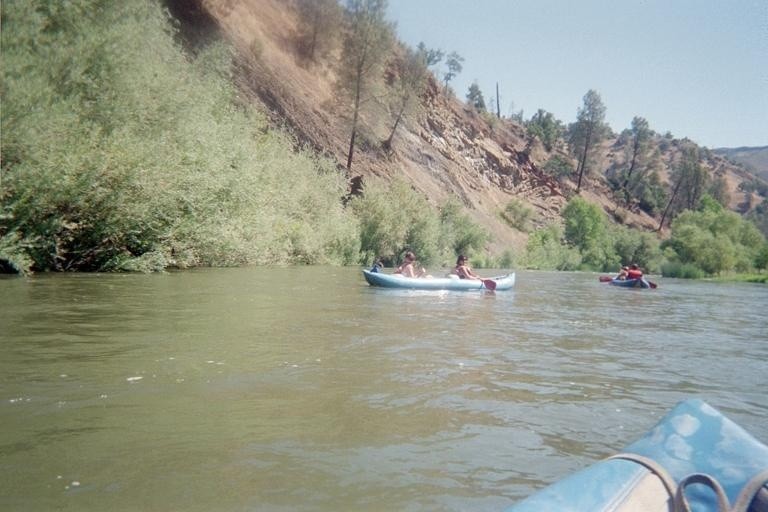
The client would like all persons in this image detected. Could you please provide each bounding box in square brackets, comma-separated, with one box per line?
[454, 255, 480, 280]
[618, 266, 629, 279]
[626, 264, 643, 280]
[399, 251, 425, 278]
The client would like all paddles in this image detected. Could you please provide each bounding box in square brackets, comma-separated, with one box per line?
[600, 276, 616, 282]
[471, 274, 496, 290]
[621, 266, 657, 289]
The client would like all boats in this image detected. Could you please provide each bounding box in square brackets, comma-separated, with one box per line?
[361, 264, 517, 292]
[610, 276, 651, 290]
[497, 396, 766, 512]
